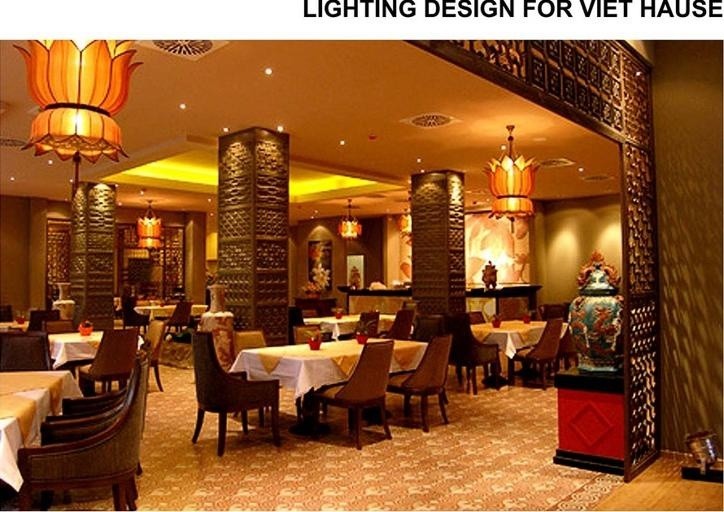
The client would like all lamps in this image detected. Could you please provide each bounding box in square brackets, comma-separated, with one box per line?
[685, 432, 719, 475]
[137, 201, 163, 257]
[13, 40, 144, 189]
[484, 125, 540, 233]
[340, 199, 358, 239]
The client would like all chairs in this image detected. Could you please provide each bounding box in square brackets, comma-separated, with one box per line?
[0, 293, 577, 511]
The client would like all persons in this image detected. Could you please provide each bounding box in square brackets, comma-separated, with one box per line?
[121, 286, 149, 326]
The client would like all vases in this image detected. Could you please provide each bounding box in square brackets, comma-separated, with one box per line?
[198, 285, 235, 372]
[53, 283, 76, 322]
